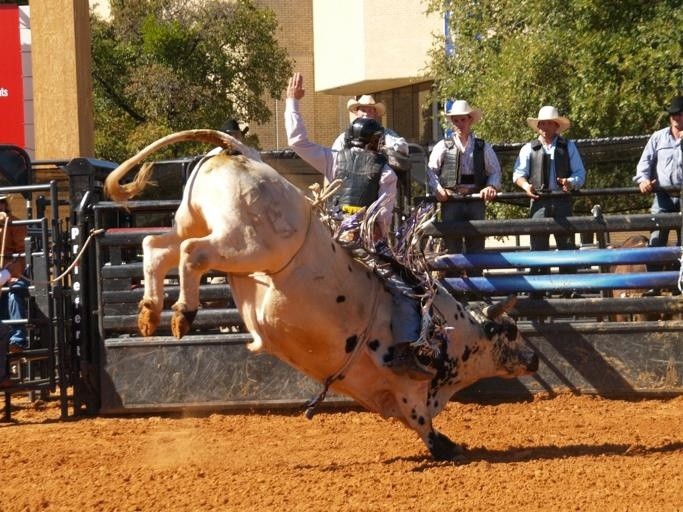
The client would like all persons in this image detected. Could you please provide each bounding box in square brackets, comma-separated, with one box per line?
[631, 94, 682, 298]
[281, 70, 435, 382]
[0, 194, 28, 354]
[511, 104, 586, 299]
[427, 99, 503, 302]
[322, 92, 409, 155]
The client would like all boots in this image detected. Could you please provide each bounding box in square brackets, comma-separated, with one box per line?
[391, 342, 434, 382]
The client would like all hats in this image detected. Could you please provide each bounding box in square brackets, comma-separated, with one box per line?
[526, 106, 570, 134]
[348, 94, 386, 116]
[667, 95, 683, 115]
[441, 100, 483, 122]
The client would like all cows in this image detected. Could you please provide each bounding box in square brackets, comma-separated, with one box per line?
[103, 129, 540, 465]
[606, 229, 683, 322]
[205, 275, 242, 333]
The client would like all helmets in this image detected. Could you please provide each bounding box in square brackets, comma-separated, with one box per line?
[348, 117, 382, 147]
